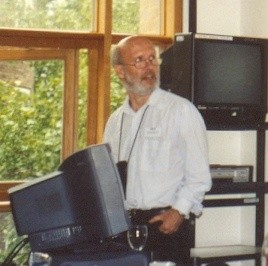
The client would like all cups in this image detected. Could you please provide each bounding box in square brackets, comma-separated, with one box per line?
[127, 225, 147, 252]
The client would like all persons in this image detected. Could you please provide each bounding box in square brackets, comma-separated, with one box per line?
[100, 35, 213, 266]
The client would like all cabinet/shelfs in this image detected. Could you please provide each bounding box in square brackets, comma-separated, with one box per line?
[187, 109, 268, 266]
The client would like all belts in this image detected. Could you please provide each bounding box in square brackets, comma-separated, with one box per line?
[129, 208, 170, 214]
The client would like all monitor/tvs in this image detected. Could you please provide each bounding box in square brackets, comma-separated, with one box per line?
[7, 143, 133, 253]
[158, 31, 268, 129]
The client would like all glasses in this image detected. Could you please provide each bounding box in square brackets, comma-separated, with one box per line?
[118, 58, 162, 68]
